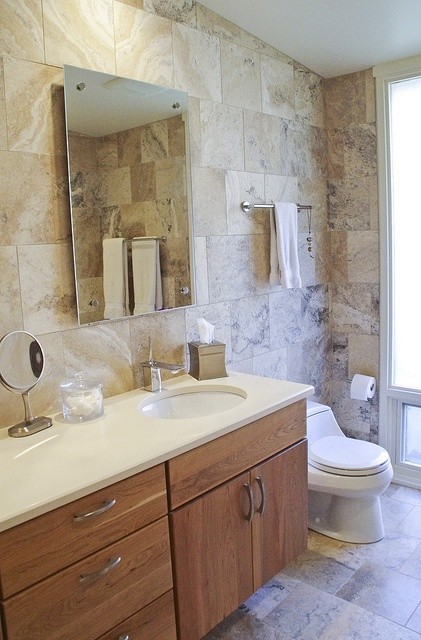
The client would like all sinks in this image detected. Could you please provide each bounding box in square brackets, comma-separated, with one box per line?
[142, 385, 248, 422]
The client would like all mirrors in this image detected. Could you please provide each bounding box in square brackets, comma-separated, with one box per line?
[63, 63, 197, 326]
[0, 330, 53, 439]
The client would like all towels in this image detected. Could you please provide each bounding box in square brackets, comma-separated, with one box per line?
[269, 201, 303, 291]
[102, 237, 133, 319]
[131, 235, 163, 316]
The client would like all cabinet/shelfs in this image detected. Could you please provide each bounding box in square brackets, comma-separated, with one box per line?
[0, 462, 179, 640]
[166, 396, 309, 640]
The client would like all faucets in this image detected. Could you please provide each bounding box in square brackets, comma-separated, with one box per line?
[140, 362, 187, 391]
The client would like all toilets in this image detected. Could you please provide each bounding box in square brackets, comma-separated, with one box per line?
[305, 400, 395, 545]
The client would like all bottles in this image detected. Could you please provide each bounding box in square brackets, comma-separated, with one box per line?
[60, 370, 105, 424]
